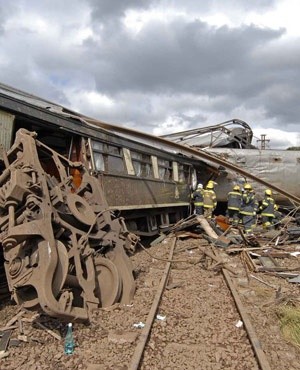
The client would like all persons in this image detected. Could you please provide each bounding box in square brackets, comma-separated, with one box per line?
[202, 181, 217, 219]
[273, 204, 283, 224]
[225, 185, 242, 225]
[257, 189, 276, 226]
[240, 182, 258, 229]
[188, 183, 204, 215]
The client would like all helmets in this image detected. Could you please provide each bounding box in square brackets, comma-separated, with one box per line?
[208, 180, 218, 185]
[233, 186, 240, 191]
[197, 183, 204, 189]
[243, 184, 252, 190]
[206, 183, 214, 189]
[265, 189, 272, 196]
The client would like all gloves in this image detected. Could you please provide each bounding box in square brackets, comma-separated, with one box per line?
[253, 212, 256, 217]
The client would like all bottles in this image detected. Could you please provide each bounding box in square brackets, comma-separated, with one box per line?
[64, 323, 74, 353]
